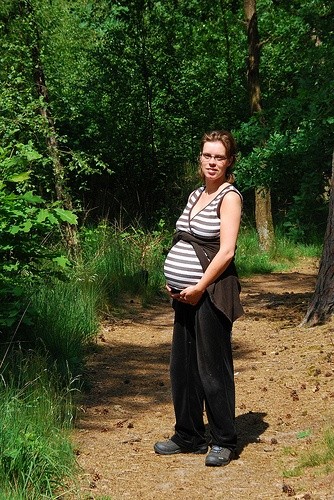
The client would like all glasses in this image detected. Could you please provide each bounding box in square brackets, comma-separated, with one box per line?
[200, 153, 228, 163]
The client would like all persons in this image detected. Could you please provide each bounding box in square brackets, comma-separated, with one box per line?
[151, 130, 246, 468]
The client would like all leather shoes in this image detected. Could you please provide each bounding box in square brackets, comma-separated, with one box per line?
[154, 439, 207, 454]
[206, 445, 232, 465]
